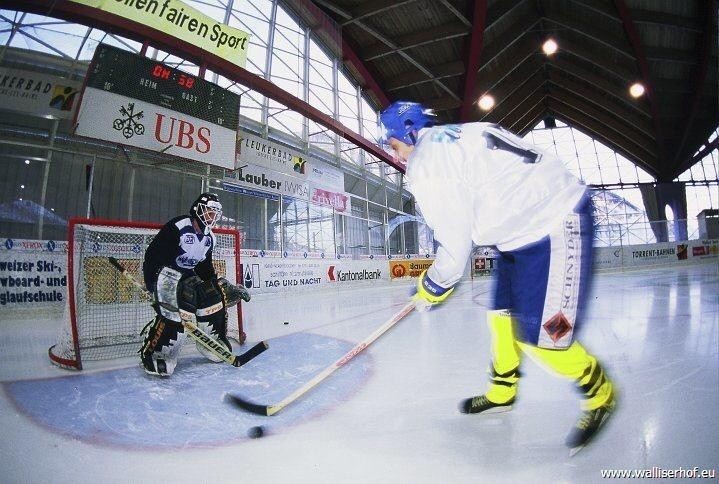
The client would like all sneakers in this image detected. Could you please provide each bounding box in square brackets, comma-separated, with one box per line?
[457, 393, 518, 415]
[563, 388, 615, 449]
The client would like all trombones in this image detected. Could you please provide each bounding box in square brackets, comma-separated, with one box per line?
[108, 254, 269, 368]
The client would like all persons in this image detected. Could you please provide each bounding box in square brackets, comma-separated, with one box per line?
[137, 191, 252, 376]
[378, 100, 617, 450]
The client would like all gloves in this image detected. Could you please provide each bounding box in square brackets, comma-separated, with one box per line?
[408, 268, 457, 313]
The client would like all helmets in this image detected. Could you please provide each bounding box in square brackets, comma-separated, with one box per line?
[189, 192, 223, 236]
[377, 99, 439, 147]
